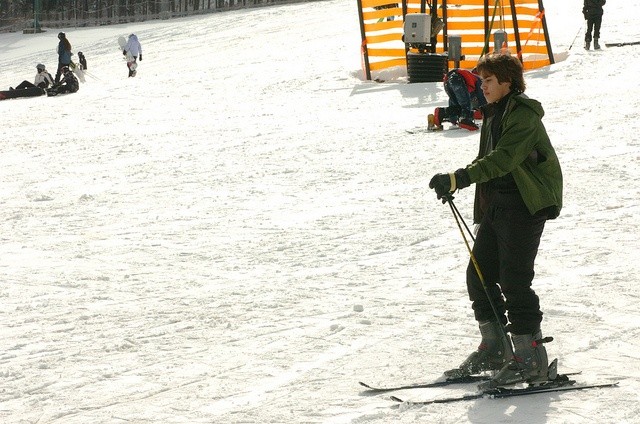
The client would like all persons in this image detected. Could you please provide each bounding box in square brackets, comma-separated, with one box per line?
[55, 32, 71, 83]
[434, 67, 486, 131]
[429, 51, 563, 392]
[8, 64, 55, 90]
[582, 0, 607, 50]
[46, 65, 79, 96]
[122, 34, 142, 77]
[78, 51, 87, 70]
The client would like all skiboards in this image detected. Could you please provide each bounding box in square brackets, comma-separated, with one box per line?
[359, 371, 619, 404]
[605, 41, 640, 48]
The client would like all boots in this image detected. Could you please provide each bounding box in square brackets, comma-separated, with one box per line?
[493, 332, 549, 385]
[459, 323, 512, 371]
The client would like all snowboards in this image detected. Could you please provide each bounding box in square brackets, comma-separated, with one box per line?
[118, 37, 137, 71]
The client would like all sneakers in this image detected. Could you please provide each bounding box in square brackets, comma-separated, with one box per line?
[434, 107, 445, 126]
[458, 119, 479, 131]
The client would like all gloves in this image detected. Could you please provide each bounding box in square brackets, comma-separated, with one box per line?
[428, 168, 471, 199]
[441, 194, 455, 203]
